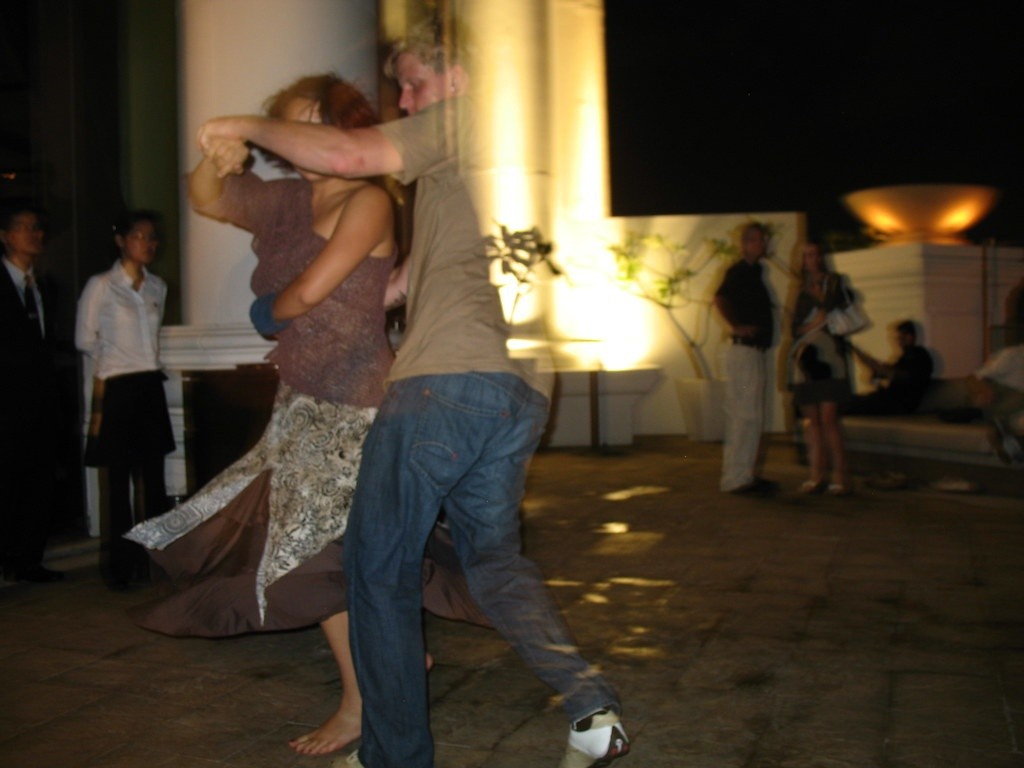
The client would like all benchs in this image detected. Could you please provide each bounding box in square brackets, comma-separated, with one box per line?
[793, 378, 1023, 474]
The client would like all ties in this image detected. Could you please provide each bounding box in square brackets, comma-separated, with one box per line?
[24, 275, 43, 340]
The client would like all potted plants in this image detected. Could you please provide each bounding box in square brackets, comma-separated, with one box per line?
[610, 229, 738, 444]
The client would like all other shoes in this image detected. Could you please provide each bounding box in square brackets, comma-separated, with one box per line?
[9, 562, 63, 582]
[737, 478, 779, 496]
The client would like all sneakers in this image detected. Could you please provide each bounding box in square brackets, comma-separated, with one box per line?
[559, 709, 631, 767]
[330, 747, 368, 766]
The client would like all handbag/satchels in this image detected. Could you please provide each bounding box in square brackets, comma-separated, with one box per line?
[824, 273, 866, 334]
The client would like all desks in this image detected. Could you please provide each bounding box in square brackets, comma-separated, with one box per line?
[181, 363, 280, 500]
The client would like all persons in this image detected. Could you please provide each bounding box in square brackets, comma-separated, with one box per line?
[0, 15, 627, 767]
[711, 223, 1024, 505]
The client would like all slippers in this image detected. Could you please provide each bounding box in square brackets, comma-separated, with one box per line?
[828, 484, 847, 495]
[804, 480, 827, 492]
